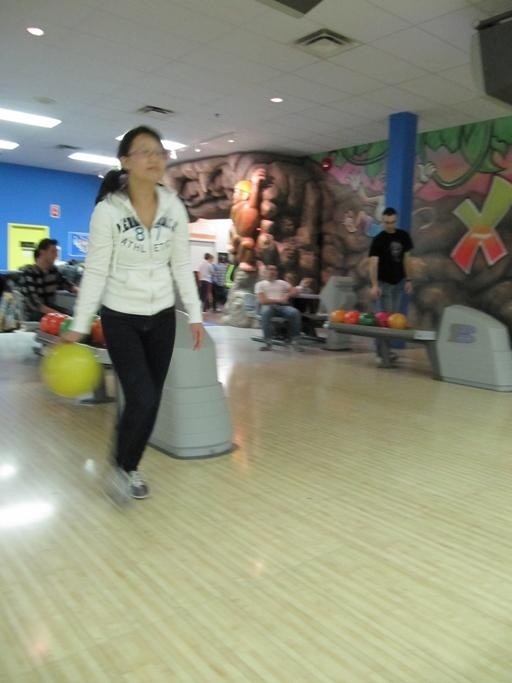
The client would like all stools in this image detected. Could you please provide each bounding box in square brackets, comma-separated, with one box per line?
[251, 315, 289, 346]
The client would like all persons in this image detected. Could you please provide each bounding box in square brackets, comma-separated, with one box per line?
[60, 259, 84, 291]
[20, 238, 80, 357]
[196, 252, 237, 314]
[255, 265, 301, 351]
[57, 126, 207, 509]
[367, 207, 415, 366]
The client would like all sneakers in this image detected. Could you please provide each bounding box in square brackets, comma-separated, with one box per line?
[95, 465, 149, 513]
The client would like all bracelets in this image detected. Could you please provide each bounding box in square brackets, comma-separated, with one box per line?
[405, 279, 412, 282]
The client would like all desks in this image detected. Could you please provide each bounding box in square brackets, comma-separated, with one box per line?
[291, 293, 328, 344]
